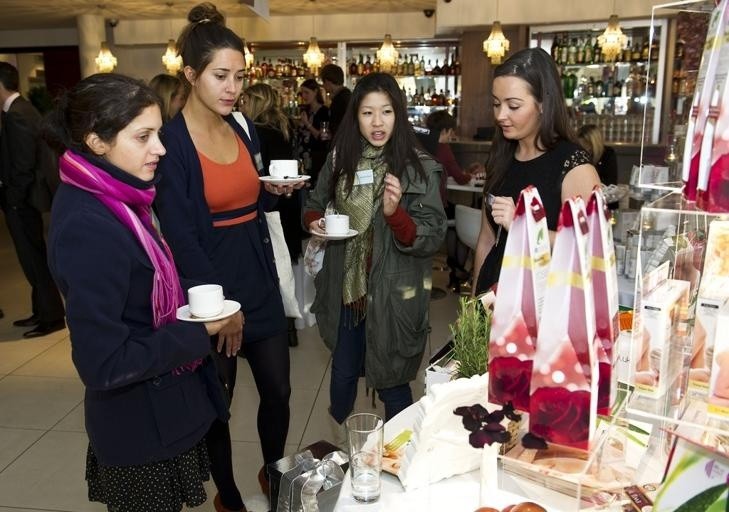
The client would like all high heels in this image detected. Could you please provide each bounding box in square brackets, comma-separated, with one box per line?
[214, 489, 247, 510]
[258, 465, 282, 512]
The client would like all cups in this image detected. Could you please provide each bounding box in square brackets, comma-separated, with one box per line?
[344, 414, 385, 503]
[187, 283, 226, 316]
[320, 214, 349, 233]
[268, 158, 299, 177]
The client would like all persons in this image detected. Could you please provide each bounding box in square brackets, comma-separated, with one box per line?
[44, 71, 235, 512]
[0, 61, 66, 339]
[422, 108, 485, 290]
[455, 46, 604, 303]
[300, 72, 451, 457]
[574, 121, 620, 209]
[148, 71, 187, 118]
[242, 65, 353, 265]
[150, 1, 308, 510]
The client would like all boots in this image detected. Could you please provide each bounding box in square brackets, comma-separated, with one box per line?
[328, 410, 354, 455]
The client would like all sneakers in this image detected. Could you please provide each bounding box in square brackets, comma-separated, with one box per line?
[459, 282, 474, 294]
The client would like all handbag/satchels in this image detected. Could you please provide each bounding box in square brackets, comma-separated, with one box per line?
[265, 211, 302, 320]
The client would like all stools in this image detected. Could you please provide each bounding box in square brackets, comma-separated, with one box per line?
[264, 438, 351, 512]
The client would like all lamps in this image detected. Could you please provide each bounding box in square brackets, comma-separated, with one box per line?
[303, 1, 325, 75]
[375, 3, 398, 72]
[480, 1, 510, 64]
[92, 0, 118, 73]
[161, 1, 182, 73]
[593, 0, 630, 68]
[233, 1, 254, 71]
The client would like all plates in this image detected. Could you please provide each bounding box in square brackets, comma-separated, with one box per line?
[309, 229, 360, 241]
[175, 299, 242, 322]
[259, 174, 311, 185]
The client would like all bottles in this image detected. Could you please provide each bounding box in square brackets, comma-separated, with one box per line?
[247, 55, 327, 76]
[558, 65, 696, 98]
[273, 84, 306, 112]
[566, 110, 688, 143]
[348, 53, 460, 76]
[402, 85, 459, 106]
[548, 32, 686, 63]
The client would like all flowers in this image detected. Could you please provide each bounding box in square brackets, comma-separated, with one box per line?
[684, 150, 729, 216]
[486, 355, 611, 452]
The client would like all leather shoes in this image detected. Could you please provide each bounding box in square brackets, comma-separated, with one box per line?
[23, 324, 65, 338]
[13, 314, 38, 326]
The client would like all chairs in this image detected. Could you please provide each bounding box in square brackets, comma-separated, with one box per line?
[432, 217, 456, 300]
[445, 203, 484, 295]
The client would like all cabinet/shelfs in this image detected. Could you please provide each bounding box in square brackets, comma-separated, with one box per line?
[241, 39, 343, 118]
[525, 16, 667, 141]
[343, 34, 464, 136]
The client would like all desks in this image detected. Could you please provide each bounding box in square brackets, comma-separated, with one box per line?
[330, 362, 728, 512]
